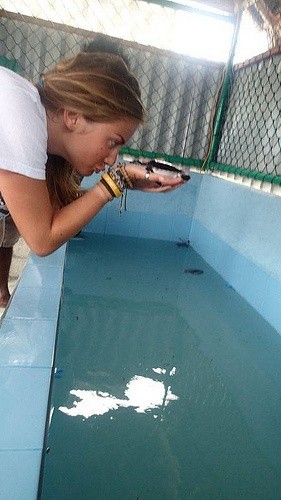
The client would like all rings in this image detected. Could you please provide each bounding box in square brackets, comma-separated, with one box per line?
[144, 172, 150, 184]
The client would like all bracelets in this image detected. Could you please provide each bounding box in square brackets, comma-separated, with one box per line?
[97, 162, 135, 203]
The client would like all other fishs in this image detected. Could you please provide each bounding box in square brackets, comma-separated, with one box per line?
[128, 158, 190, 185]
[184, 269, 203, 276]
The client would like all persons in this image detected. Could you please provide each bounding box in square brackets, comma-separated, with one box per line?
[0, 51, 191, 257]
[0, 29, 132, 310]
